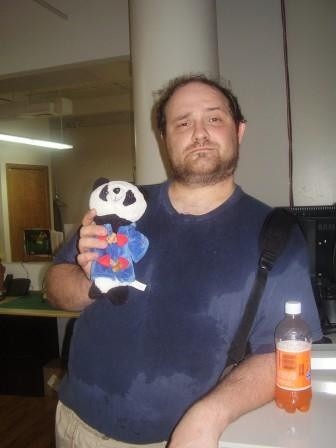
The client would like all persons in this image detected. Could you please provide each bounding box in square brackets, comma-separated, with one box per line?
[42, 72, 323, 448]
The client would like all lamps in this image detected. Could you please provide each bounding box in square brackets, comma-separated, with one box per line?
[0, 115, 74, 149]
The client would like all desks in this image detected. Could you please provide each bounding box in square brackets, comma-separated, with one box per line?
[217, 343, 336, 448]
[0, 291, 81, 377]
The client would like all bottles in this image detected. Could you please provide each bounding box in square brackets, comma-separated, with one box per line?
[273, 300, 312, 413]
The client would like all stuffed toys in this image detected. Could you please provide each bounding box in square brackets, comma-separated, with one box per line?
[84, 177, 150, 308]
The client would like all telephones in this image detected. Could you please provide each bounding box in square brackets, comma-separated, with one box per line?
[4, 273, 32, 296]
